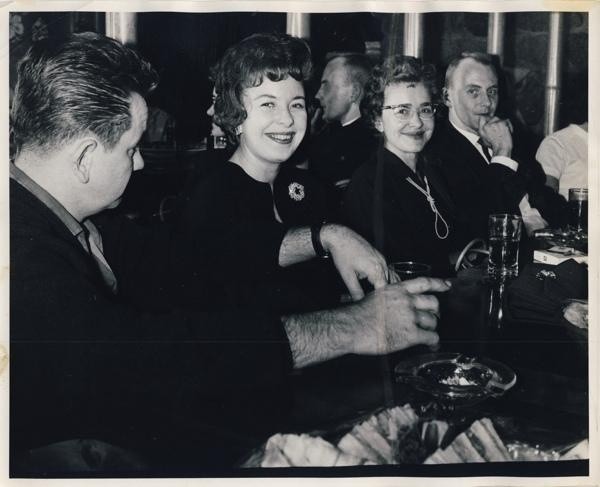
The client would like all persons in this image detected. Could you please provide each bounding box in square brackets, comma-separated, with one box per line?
[176, 31, 546, 387]
[535, 122, 588, 201]
[10, 32, 454, 443]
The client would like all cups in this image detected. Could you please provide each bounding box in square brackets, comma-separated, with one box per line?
[387, 263, 430, 282]
[487, 215, 521, 281]
[568, 188, 588, 241]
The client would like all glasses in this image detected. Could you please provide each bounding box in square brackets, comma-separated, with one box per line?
[379, 102, 437, 120]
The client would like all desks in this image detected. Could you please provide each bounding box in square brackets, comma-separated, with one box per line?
[230, 228, 588, 472]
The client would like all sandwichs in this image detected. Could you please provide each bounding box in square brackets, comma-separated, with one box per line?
[336, 403, 514, 464]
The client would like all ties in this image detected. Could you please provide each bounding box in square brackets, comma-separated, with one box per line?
[478, 135, 499, 164]
